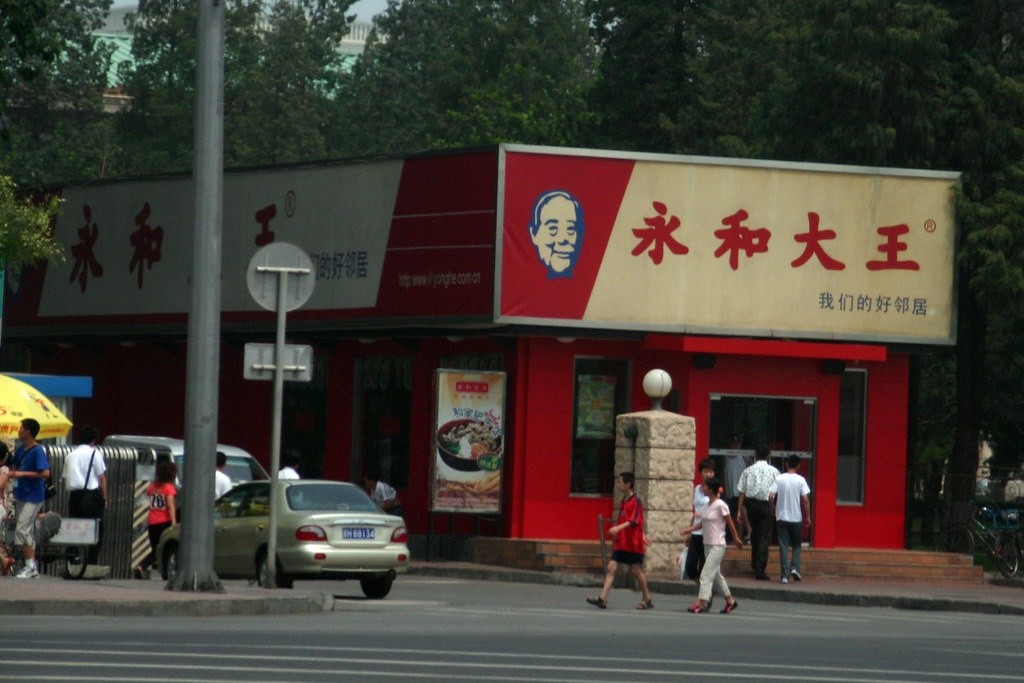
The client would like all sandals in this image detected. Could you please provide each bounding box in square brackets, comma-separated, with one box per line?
[636, 600, 654, 609]
[587, 596, 607, 609]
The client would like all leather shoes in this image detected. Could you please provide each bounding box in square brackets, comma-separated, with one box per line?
[756, 571, 770, 580]
[688, 601, 710, 613]
[720, 600, 737, 613]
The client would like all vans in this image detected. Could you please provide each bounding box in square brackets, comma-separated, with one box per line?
[102, 431, 269, 515]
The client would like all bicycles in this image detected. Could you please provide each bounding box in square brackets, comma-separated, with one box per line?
[936, 499, 1024, 581]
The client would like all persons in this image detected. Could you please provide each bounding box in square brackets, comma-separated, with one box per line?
[215, 451, 232, 501]
[9, 419, 50, 578]
[679, 478, 744, 614]
[769, 455, 811, 583]
[718, 440, 752, 544]
[63, 425, 109, 566]
[0, 442, 13, 576]
[737, 444, 781, 580]
[134, 461, 178, 580]
[586, 473, 654, 610]
[685, 458, 724, 608]
[278, 456, 301, 479]
[364, 472, 404, 515]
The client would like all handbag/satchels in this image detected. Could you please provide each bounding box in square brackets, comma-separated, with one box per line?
[44, 474, 57, 500]
[70, 492, 87, 515]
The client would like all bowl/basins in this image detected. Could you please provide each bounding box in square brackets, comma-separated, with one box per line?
[436, 420, 502, 472]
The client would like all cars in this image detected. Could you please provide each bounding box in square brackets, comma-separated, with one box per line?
[154, 477, 411, 599]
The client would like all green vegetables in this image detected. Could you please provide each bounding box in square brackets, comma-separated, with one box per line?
[441, 441, 460, 454]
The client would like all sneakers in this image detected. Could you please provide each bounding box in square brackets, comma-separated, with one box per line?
[791, 570, 802, 580]
[16, 566, 40, 578]
[782, 578, 789, 583]
[135, 566, 144, 580]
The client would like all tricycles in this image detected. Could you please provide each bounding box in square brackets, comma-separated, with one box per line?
[0, 489, 103, 581]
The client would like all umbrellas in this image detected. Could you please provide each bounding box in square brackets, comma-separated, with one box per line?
[0, 372, 73, 440]
